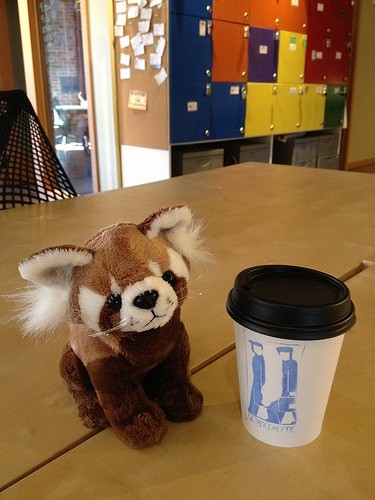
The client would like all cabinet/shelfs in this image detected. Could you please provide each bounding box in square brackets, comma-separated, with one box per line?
[169, 0, 360, 145]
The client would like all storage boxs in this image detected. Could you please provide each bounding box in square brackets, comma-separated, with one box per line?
[175, 132, 341, 175]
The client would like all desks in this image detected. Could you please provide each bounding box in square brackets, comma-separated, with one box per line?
[0, 161, 375, 499]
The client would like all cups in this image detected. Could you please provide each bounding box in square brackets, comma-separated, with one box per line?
[225, 264, 357, 448]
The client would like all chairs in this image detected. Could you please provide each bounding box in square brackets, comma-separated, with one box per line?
[0, 89, 78, 210]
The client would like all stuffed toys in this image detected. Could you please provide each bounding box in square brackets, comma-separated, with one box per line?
[5, 202, 215, 452]
